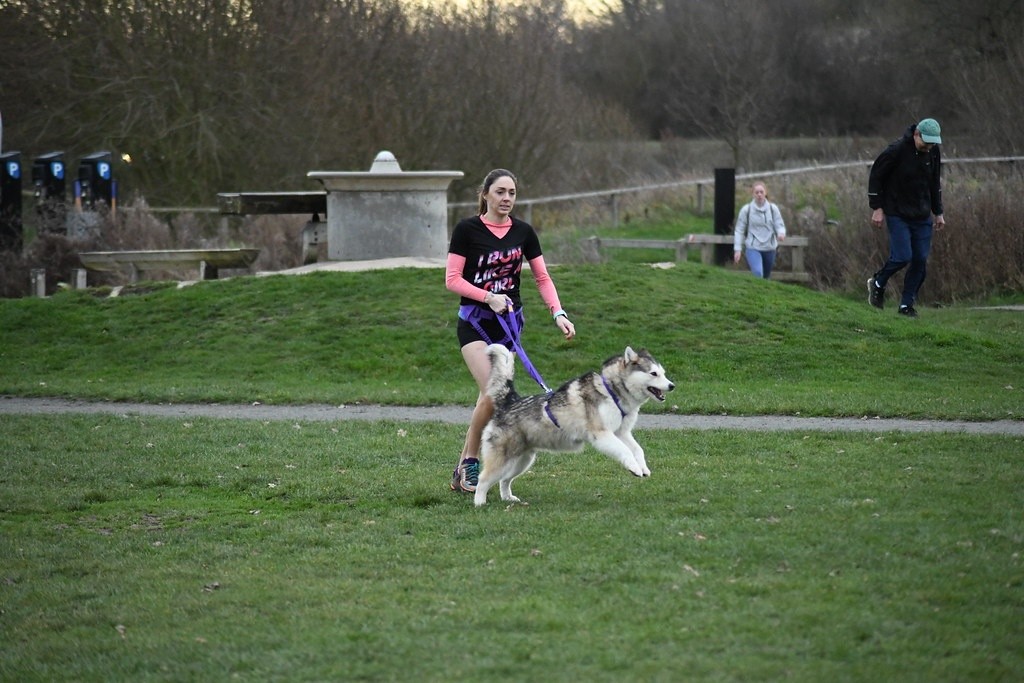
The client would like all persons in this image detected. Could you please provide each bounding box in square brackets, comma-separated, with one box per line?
[734, 182, 786, 281]
[867, 119, 945, 320]
[444, 170, 576, 493]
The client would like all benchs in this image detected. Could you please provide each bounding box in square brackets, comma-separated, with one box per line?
[78, 247, 259, 285]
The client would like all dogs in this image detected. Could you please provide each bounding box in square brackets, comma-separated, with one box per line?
[473, 343, 675, 508]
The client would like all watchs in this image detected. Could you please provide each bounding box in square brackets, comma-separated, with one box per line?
[553, 310, 568, 320]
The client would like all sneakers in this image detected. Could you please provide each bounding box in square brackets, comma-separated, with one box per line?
[867, 277, 886, 311]
[450, 465, 461, 491]
[898, 304, 920, 319]
[459, 457, 480, 492]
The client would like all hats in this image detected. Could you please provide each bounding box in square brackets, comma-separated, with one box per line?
[916, 118, 942, 145]
